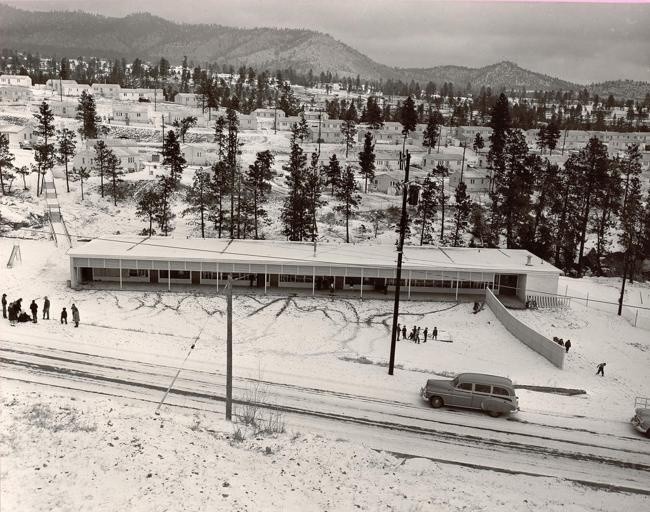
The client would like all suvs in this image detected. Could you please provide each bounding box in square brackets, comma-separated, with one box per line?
[422, 373, 519, 417]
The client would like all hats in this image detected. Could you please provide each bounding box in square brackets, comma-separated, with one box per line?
[3, 293, 7, 297]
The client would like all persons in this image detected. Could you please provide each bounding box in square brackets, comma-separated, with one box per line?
[73, 307, 80, 327]
[71, 303, 76, 321]
[396, 323, 439, 344]
[558, 339, 564, 346]
[60, 308, 67, 324]
[565, 339, 571, 352]
[0, 293, 51, 327]
[596, 362, 606, 377]
[525, 300, 538, 310]
